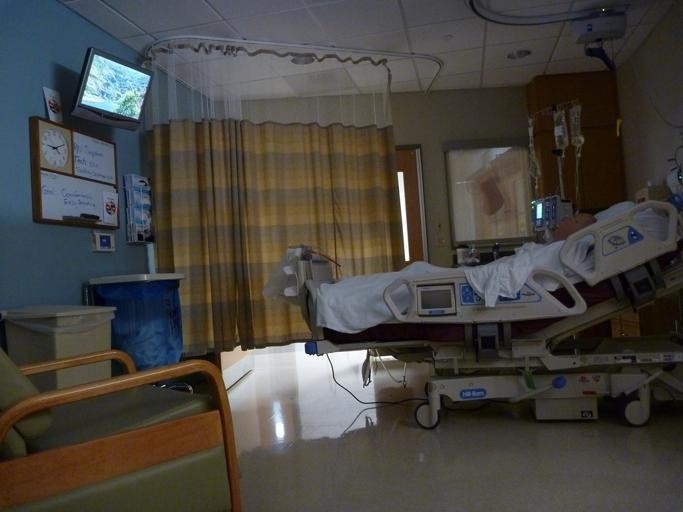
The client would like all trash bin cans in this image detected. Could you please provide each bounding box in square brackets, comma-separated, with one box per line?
[0, 305, 116, 392]
[179, 353, 216, 394]
[84, 273, 185, 376]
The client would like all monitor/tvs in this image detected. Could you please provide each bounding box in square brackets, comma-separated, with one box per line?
[530, 195, 574, 232]
[70, 46, 155, 132]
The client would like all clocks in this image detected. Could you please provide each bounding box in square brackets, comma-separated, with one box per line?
[41, 128, 69, 167]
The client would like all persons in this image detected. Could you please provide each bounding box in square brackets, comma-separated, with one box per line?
[548, 212, 597, 242]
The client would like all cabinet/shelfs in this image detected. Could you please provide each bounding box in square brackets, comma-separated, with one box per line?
[527, 70, 627, 242]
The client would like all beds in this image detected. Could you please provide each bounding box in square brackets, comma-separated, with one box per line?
[287, 203, 679, 429]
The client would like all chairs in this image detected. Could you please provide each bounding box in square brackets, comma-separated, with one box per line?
[0, 350, 241, 511]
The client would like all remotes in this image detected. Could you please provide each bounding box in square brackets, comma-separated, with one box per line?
[81, 214, 99, 219]
